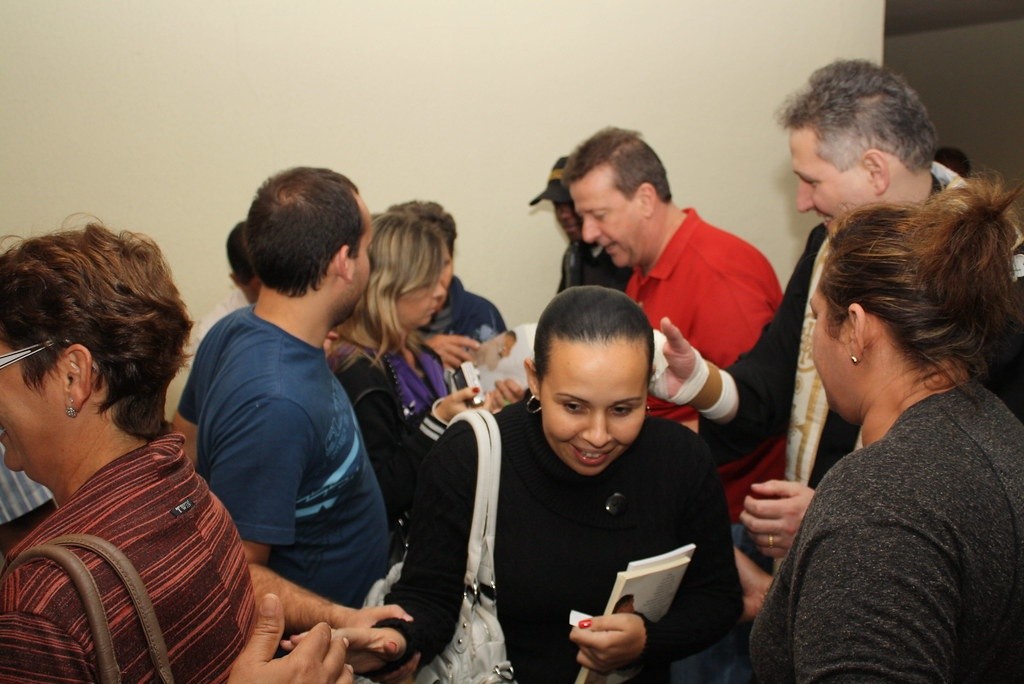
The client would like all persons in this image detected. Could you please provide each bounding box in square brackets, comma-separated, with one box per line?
[558, 125, 791, 573]
[279, 285, 742, 684]
[651, 59, 1023, 577]
[745, 175, 1024, 684]
[932, 143, 972, 178]
[0, 223, 353, 684]
[612, 594, 635, 614]
[170, 166, 508, 684]
[529, 156, 635, 292]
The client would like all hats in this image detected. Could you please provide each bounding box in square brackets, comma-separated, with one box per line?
[529, 156, 572, 206]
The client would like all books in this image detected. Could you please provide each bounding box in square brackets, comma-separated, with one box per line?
[458, 322, 537, 407]
[575, 543, 696, 684]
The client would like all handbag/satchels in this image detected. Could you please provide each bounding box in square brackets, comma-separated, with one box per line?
[359, 405, 516, 684]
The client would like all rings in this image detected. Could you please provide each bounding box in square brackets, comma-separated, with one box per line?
[769, 535, 773, 549]
